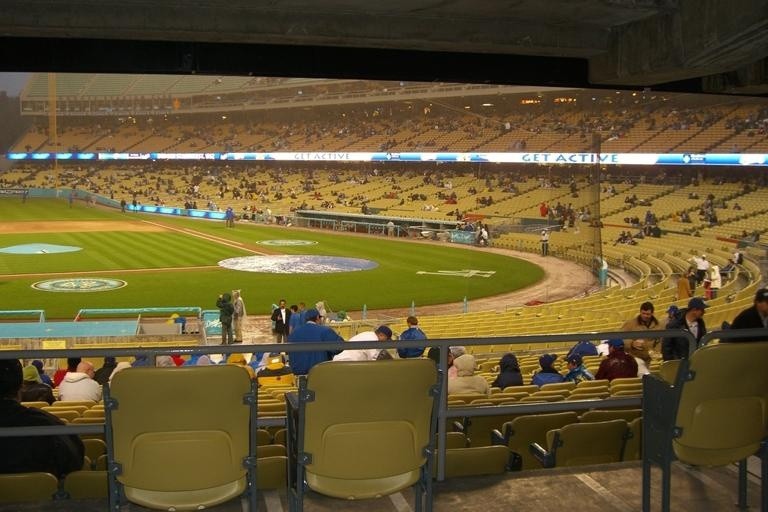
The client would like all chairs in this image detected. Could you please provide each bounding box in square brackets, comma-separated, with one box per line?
[596, 67, 767, 509]
[3, 298, 593, 510]
[298, 68, 621, 272]
[4, 71, 297, 221]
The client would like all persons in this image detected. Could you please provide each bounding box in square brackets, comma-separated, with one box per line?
[157, 352, 291, 383]
[428, 296, 711, 394]
[1, 94, 767, 300]
[718, 288, 768, 343]
[270, 300, 428, 375]
[165, 313, 186, 330]
[216, 290, 248, 345]
[0, 356, 145, 473]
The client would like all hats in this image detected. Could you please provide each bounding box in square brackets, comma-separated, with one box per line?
[539, 354, 580, 365]
[688, 297, 710, 308]
[22, 365, 39, 382]
[756, 289, 768, 302]
[304, 309, 322, 320]
[378, 326, 393, 340]
[604, 339, 624, 347]
